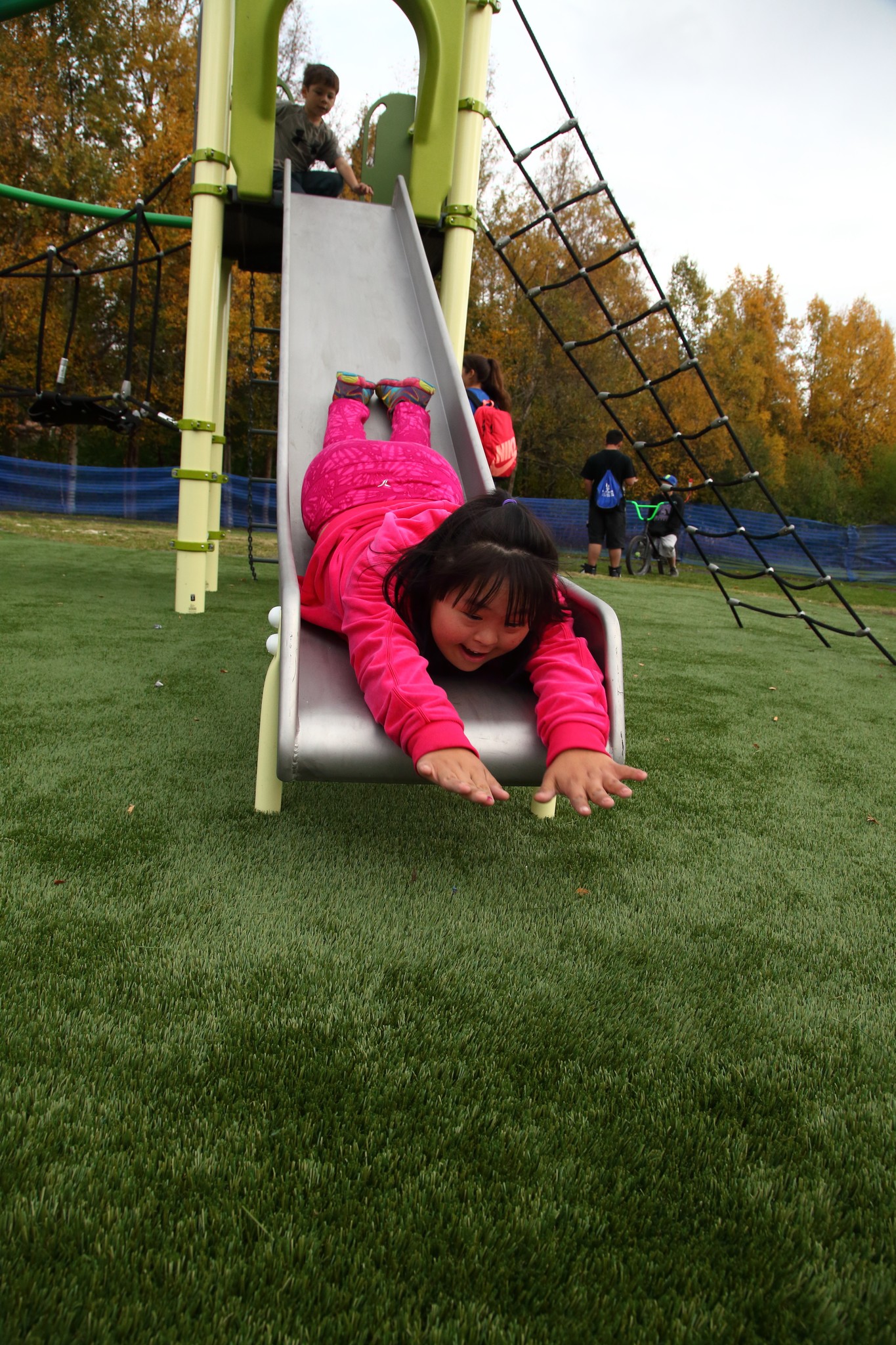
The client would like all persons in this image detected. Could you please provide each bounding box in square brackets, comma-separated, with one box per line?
[297, 371, 648, 817]
[578, 430, 637, 578]
[463, 354, 517, 482]
[638, 474, 683, 576]
[274, 63, 377, 200]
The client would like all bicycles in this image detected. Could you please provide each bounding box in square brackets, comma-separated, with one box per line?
[626, 500, 681, 577]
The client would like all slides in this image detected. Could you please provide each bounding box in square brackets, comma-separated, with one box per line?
[252, 152, 641, 820]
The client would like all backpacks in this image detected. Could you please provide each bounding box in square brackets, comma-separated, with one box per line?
[466, 388, 517, 482]
[593, 452, 625, 512]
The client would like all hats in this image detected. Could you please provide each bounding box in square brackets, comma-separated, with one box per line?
[659, 473, 677, 487]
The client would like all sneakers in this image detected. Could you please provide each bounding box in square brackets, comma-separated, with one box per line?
[332, 371, 376, 406]
[375, 377, 436, 422]
[607, 565, 622, 579]
[579, 563, 598, 576]
[668, 565, 679, 578]
